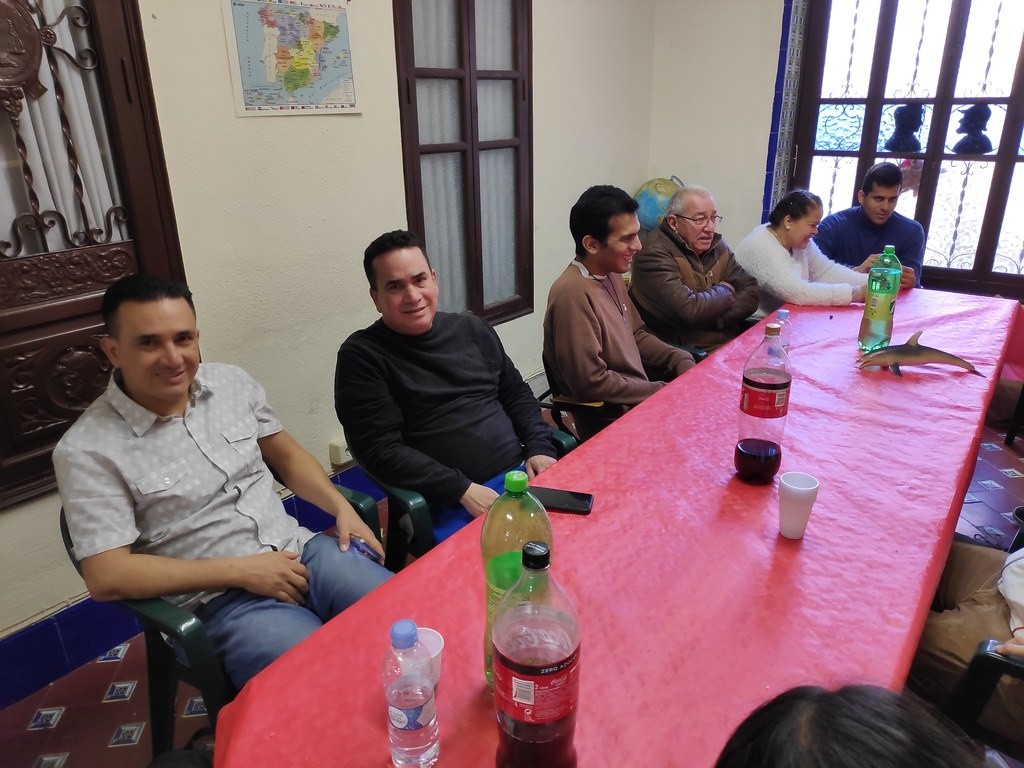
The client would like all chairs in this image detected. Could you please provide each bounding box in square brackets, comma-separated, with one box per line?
[60, 288, 761, 762]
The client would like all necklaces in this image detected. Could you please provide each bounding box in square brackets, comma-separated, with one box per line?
[773, 229, 791, 250]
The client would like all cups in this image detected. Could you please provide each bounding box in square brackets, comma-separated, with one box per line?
[777, 471, 819, 539]
[409, 628, 445, 686]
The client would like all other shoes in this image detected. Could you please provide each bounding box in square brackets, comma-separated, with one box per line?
[1013, 506, 1024, 525]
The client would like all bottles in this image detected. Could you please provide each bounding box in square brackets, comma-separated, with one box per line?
[480, 473, 555, 694]
[490, 541, 581, 768]
[379, 620, 441, 767]
[732, 322, 793, 483]
[857, 245, 903, 353]
[767, 308, 792, 366]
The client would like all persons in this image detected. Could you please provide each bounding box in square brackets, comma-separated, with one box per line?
[884, 105, 925, 151]
[733, 190, 869, 328]
[951, 104, 992, 154]
[335, 229, 559, 545]
[542, 185, 696, 444]
[627, 185, 762, 356]
[52, 273, 395, 691]
[813, 162, 926, 289]
[917, 542, 1024, 746]
[714, 684, 997, 768]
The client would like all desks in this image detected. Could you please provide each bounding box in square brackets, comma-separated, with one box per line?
[216, 290, 1020, 768]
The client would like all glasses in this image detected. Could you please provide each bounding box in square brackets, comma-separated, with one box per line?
[674, 214, 723, 226]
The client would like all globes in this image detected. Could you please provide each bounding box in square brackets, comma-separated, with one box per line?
[634, 175, 686, 235]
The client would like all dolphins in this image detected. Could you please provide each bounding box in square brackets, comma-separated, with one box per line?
[856, 330, 985, 377]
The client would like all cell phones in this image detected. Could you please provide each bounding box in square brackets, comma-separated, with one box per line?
[526, 486, 594, 516]
[332, 530, 382, 562]
